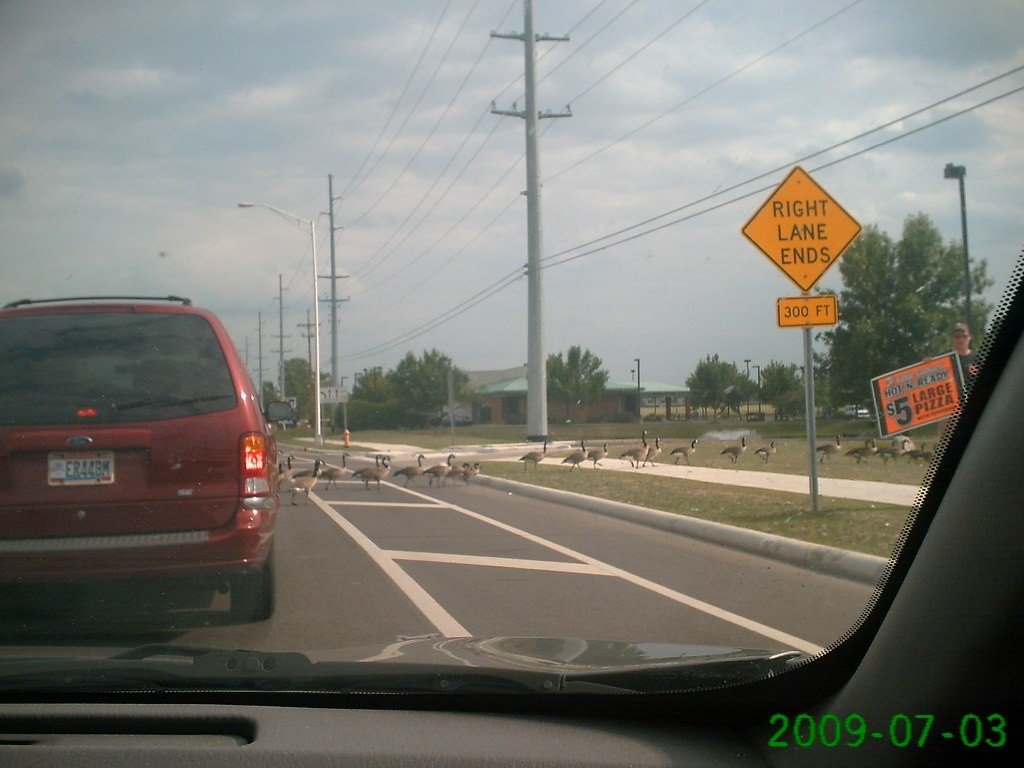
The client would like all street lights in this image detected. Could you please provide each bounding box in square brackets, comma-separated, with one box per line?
[944, 163, 974, 350]
[238, 200, 323, 444]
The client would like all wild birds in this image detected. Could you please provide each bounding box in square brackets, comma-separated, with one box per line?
[642, 436, 662, 467]
[872, 438, 938, 467]
[423, 453, 457, 488]
[318, 452, 352, 491]
[277, 450, 298, 503]
[442, 462, 482, 488]
[588, 442, 608, 469]
[561, 439, 589, 472]
[519, 438, 553, 471]
[753, 440, 777, 465]
[669, 439, 700, 466]
[393, 454, 426, 488]
[817, 434, 842, 464]
[288, 458, 327, 505]
[720, 436, 747, 465]
[618, 429, 650, 468]
[843, 438, 879, 465]
[353, 454, 392, 491]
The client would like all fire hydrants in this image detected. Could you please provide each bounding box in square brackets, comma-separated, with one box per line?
[343, 429, 350, 448]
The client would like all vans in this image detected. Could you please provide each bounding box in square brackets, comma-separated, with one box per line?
[0, 292, 294, 620]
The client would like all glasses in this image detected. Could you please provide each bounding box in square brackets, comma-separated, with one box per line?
[953, 333, 968, 339]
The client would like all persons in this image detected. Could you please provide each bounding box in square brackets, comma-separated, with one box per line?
[923, 322, 980, 385]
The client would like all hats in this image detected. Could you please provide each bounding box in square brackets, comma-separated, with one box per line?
[952, 322, 969, 332]
